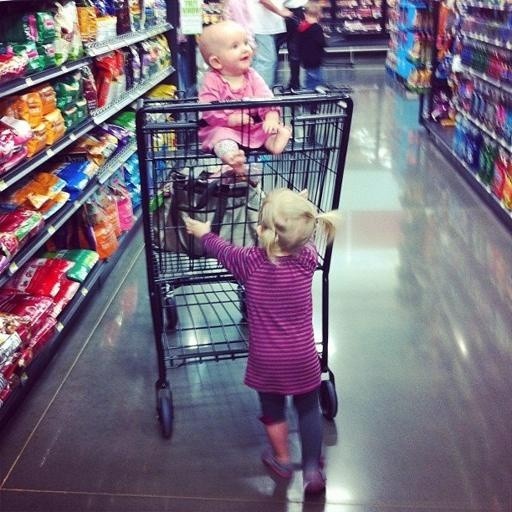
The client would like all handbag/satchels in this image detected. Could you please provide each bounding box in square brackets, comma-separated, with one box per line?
[161, 170, 266, 256]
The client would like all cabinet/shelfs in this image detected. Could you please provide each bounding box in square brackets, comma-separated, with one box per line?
[324, 0, 433, 95]
[0, 0, 197, 438]
[418, 0, 512, 234]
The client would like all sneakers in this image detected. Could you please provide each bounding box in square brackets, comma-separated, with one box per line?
[261, 448, 292, 479]
[304, 471, 326, 497]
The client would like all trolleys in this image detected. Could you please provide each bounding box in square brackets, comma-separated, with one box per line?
[135, 83, 353, 438]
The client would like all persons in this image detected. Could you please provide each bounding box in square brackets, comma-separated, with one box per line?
[300, 4, 325, 86]
[188, 187, 346, 493]
[245, 0, 294, 89]
[281, 0, 312, 93]
[197, 21, 293, 175]
